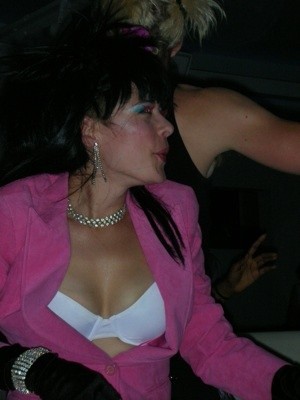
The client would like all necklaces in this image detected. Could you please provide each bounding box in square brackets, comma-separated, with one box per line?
[65, 202, 128, 228]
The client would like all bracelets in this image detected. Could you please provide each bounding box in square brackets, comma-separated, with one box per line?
[215, 285, 231, 300]
[11, 346, 49, 395]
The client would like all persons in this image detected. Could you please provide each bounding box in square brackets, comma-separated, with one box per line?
[0, 31, 300, 400]
[76, 0, 300, 330]
